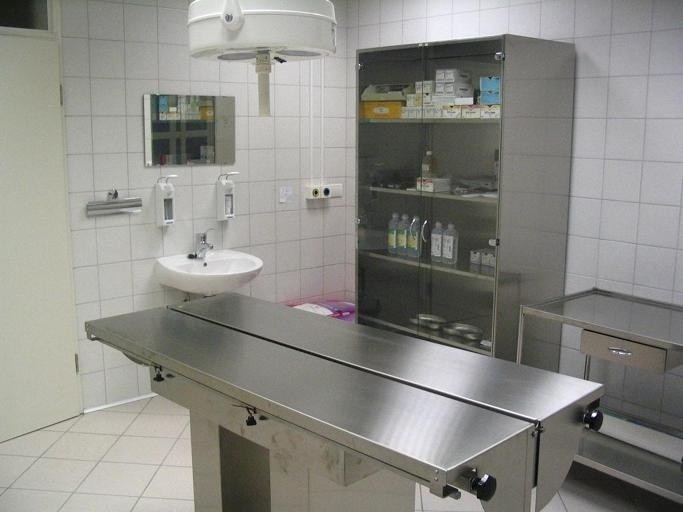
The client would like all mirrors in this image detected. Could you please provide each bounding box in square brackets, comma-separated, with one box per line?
[144, 94, 235, 166]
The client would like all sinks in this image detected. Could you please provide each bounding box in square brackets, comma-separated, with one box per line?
[154, 250, 264, 295]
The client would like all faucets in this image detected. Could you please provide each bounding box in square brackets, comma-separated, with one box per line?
[196, 227, 217, 257]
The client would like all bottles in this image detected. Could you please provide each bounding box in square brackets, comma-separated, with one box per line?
[421, 150, 436, 179]
[407, 215, 423, 258]
[430, 221, 445, 263]
[396, 214, 411, 257]
[387, 211, 399, 254]
[441, 222, 459, 266]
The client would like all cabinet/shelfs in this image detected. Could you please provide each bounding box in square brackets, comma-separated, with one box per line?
[354, 33, 574, 372]
[516, 286, 683, 503]
[150, 94, 215, 166]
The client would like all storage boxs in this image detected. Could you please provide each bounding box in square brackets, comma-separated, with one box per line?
[480, 76, 500, 105]
[360, 102, 404, 118]
